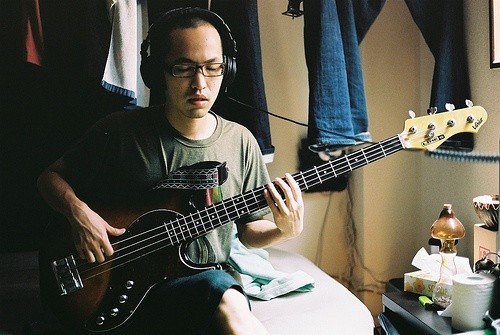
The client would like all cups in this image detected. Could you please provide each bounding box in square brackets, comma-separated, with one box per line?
[492, 319, 500, 335]
[428, 237, 458, 255]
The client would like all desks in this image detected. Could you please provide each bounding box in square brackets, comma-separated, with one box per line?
[382, 289, 465, 335]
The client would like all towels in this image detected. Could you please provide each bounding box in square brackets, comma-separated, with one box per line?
[99, 0, 152, 110]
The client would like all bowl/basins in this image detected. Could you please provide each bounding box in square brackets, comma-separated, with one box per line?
[473, 195, 499, 231]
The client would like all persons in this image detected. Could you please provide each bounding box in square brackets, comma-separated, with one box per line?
[36, 16, 304, 335]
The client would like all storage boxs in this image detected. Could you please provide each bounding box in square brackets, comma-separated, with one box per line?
[472, 224, 500, 271]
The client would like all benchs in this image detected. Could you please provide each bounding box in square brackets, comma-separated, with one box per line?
[244, 245, 376, 335]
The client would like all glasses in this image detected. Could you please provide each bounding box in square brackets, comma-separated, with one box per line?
[162, 55, 227, 78]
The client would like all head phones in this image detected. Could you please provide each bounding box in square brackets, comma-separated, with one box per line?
[141, 7, 238, 89]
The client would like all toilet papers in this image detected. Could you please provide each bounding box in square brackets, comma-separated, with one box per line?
[435, 273, 496, 332]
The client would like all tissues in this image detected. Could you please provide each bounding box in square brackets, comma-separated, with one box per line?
[402, 246, 475, 297]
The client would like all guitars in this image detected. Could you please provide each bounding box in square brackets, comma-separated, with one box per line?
[0, 98, 488, 334]
